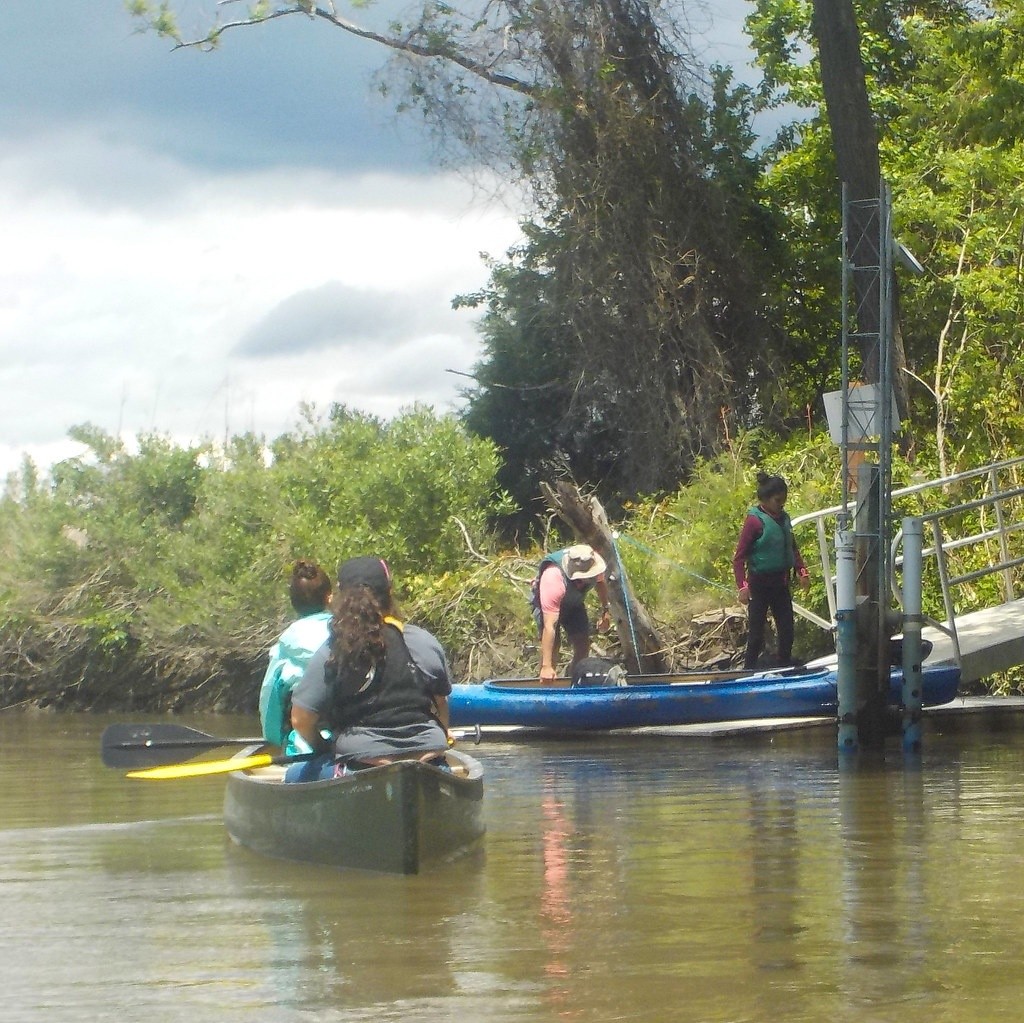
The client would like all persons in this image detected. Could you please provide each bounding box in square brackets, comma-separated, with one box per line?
[258, 556, 453, 784]
[733, 471, 812, 670]
[531, 544, 611, 685]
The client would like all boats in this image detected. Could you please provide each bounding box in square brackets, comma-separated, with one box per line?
[219, 721, 490, 878]
[444, 659, 960, 732]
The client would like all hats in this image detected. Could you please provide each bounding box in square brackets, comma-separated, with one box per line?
[561, 544, 608, 581]
[335, 557, 391, 594]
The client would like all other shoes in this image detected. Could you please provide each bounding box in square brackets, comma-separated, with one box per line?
[778, 654, 803, 667]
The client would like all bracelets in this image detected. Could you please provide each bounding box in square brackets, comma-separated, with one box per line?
[598, 603, 609, 614]
[799, 568, 810, 580]
[738, 581, 748, 592]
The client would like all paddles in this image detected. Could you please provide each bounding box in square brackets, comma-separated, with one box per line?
[123, 739, 454, 781]
[99, 721, 269, 771]
